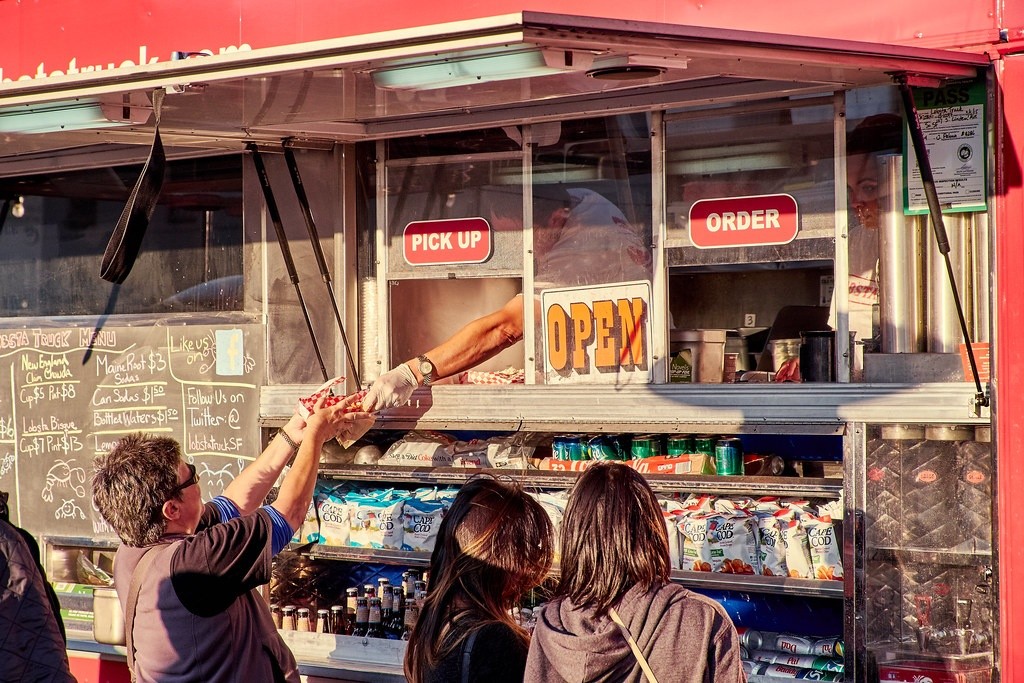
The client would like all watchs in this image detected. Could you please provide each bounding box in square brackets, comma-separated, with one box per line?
[418, 354, 433, 386]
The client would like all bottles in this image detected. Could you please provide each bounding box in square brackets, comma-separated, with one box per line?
[268, 568, 430, 641]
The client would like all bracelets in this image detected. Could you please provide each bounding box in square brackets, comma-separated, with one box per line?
[278, 428, 298, 449]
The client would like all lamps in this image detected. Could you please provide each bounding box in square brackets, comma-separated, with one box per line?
[371, 47, 596, 96]
[488, 153, 611, 187]
[665, 140, 815, 181]
[0, 92, 154, 136]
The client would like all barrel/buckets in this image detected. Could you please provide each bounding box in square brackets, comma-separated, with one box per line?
[769, 338, 799, 371]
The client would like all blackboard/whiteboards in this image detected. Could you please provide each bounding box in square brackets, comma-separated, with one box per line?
[0, 313, 261, 547]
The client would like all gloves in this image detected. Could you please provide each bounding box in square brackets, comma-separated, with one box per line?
[358, 364, 419, 411]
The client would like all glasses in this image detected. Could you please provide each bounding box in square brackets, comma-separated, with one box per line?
[164, 463, 201, 501]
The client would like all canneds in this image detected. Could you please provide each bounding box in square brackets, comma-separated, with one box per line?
[735, 627, 846, 683]
[552, 433, 745, 476]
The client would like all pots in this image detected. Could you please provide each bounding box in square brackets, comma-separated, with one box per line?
[800, 330, 857, 382]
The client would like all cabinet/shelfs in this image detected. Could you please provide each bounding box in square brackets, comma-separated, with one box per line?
[259, 418, 856, 682]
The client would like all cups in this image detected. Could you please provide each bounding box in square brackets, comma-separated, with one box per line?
[723, 352, 739, 383]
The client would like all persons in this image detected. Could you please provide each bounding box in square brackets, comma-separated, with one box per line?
[827, 112, 903, 354]
[88, 393, 371, 683]
[402, 472, 555, 683]
[0, 491, 78, 683]
[363, 184, 653, 412]
[523, 462, 748, 683]
